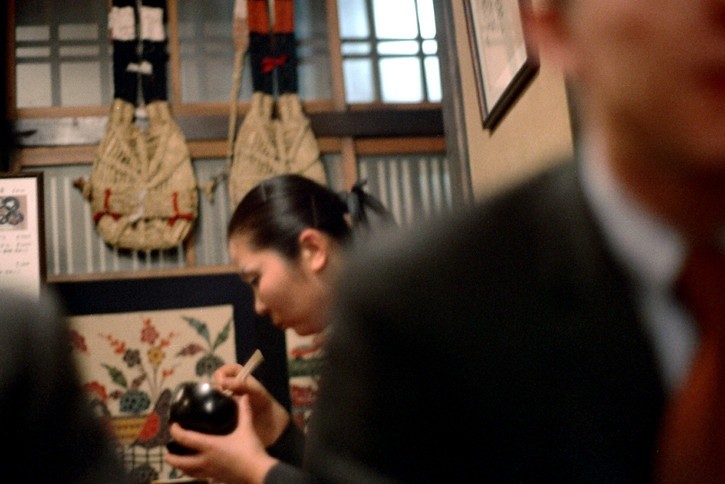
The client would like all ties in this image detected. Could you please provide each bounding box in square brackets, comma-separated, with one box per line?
[655, 249, 725, 484]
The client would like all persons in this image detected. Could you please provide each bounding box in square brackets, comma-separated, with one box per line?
[302, 0, 724, 484]
[165, 174, 397, 484]
[0, 285, 135, 484]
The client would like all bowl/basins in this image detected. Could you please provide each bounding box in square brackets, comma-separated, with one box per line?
[164, 381, 238, 456]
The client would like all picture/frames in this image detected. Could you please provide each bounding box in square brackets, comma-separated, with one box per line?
[461, 0, 538, 130]
[0, 171, 47, 302]
